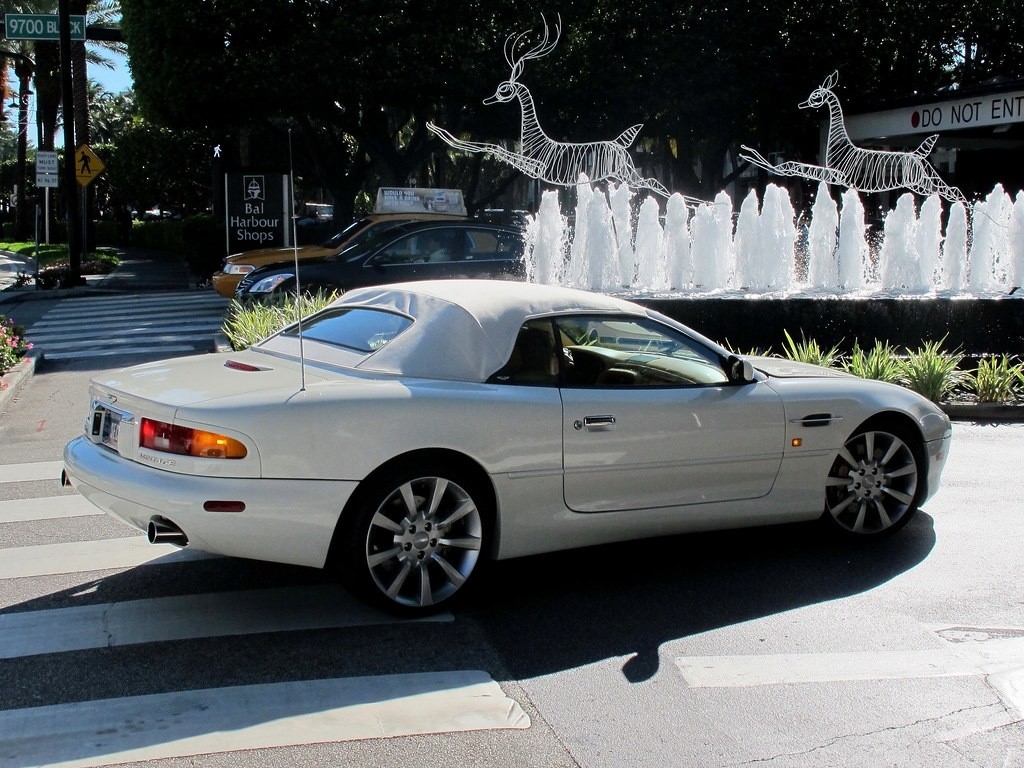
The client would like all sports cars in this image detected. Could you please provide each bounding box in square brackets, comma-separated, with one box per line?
[60, 278, 955, 621]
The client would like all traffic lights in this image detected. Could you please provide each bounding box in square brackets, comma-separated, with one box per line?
[209, 131, 239, 164]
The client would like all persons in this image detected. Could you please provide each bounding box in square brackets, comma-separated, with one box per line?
[112, 204, 124, 248]
[490, 341, 530, 385]
[122, 205, 132, 250]
[292, 205, 305, 247]
[539, 358, 580, 388]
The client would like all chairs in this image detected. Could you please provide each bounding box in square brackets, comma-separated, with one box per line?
[525, 327, 554, 375]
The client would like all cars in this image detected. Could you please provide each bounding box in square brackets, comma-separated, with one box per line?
[132, 209, 172, 221]
[474, 208, 534, 232]
[210, 186, 507, 301]
[303, 201, 333, 222]
[234, 219, 534, 310]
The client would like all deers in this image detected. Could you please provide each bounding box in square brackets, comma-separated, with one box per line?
[424, 10, 671, 198]
[738, 69, 973, 208]
